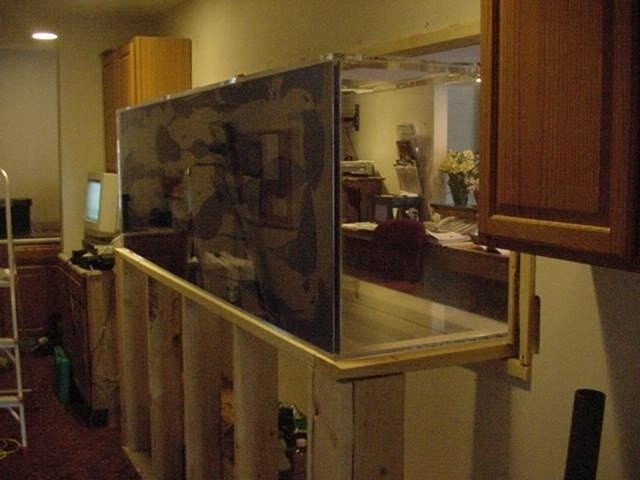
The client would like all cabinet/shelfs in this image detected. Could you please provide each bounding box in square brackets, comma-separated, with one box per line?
[474, 0, 640, 272]
[102, 36, 191, 174]
[115, 247, 511, 479]
[57, 267, 118, 427]
[0, 244, 54, 343]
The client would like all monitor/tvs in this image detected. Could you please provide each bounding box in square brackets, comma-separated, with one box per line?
[82, 170, 121, 242]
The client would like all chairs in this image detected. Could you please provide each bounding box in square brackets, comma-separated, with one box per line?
[342, 218, 427, 295]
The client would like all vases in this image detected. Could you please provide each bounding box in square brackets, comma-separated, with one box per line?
[446, 173, 469, 206]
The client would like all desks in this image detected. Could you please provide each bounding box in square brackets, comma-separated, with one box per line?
[342, 221, 508, 313]
[343, 176, 423, 219]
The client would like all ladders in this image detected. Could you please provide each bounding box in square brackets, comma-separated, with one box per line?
[0, 169, 29, 457]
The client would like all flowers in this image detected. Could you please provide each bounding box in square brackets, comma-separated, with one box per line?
[438, 148, 478, 192]
